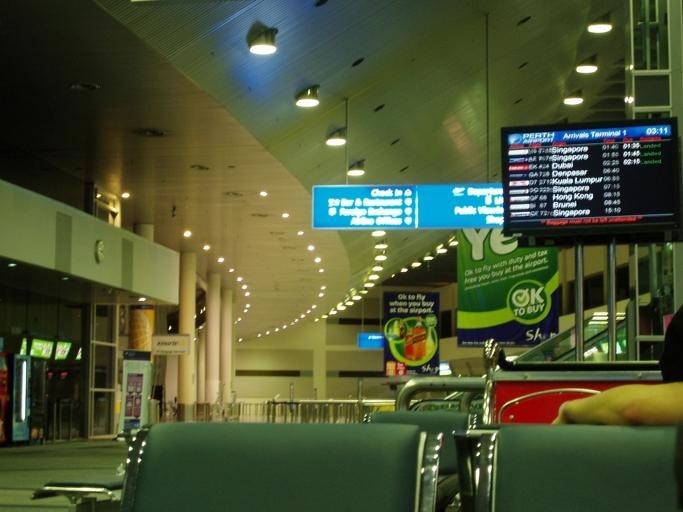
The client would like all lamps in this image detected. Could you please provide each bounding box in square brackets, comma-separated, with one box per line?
[404, 236, 460, 274]
[291, 230, 388, 323]
[576, 58, 598, 75]
[251, 30, 277, 55]
[259, 190, 268, 197]
[563, 90, 583, 106]
[296, 84, 320, 108]
[326, 128, 347, 147]
[347, 160, 365, 176]
[588, 16, 613, 34]
[281, 212, 289, 219]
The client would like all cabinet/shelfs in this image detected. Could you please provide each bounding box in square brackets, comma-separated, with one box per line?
[394, 315, 426, 361]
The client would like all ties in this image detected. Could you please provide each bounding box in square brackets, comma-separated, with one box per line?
[500, 116, 678, 234]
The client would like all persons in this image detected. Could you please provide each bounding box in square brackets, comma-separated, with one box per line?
[541, 379, 682, 430]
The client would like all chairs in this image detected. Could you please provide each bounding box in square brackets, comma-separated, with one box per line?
[31, 411, 683, 512]
